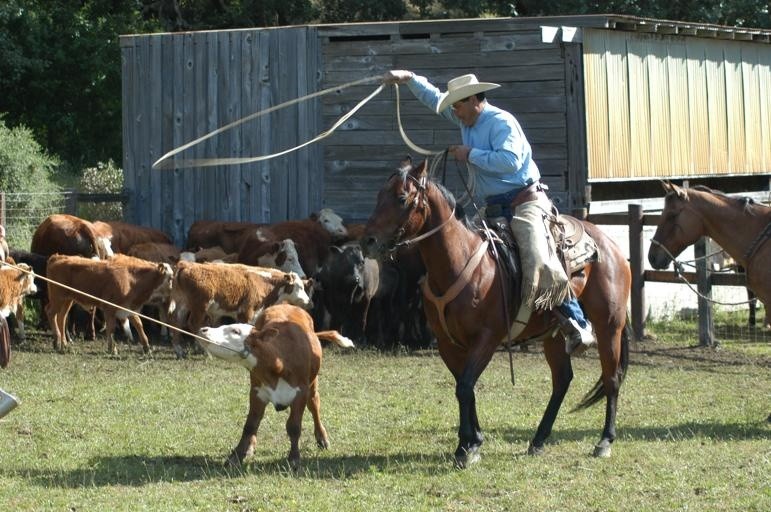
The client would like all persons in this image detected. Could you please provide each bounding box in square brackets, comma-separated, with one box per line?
[384, 68, 598, 354]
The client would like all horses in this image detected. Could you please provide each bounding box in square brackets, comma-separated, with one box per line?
[359, 153, 639, 471]
[648, 178, 771, 326]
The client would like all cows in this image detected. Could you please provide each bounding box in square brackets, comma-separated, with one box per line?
[196, 303, 356, 480]
[0, 207, 436, 361]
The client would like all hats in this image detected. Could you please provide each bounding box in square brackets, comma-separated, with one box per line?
[436, 73, 501, 115]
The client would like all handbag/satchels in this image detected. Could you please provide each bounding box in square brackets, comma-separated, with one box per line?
[485, 204, 513, 222]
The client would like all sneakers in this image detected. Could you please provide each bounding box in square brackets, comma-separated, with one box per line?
[570, 321, 594, 352]
